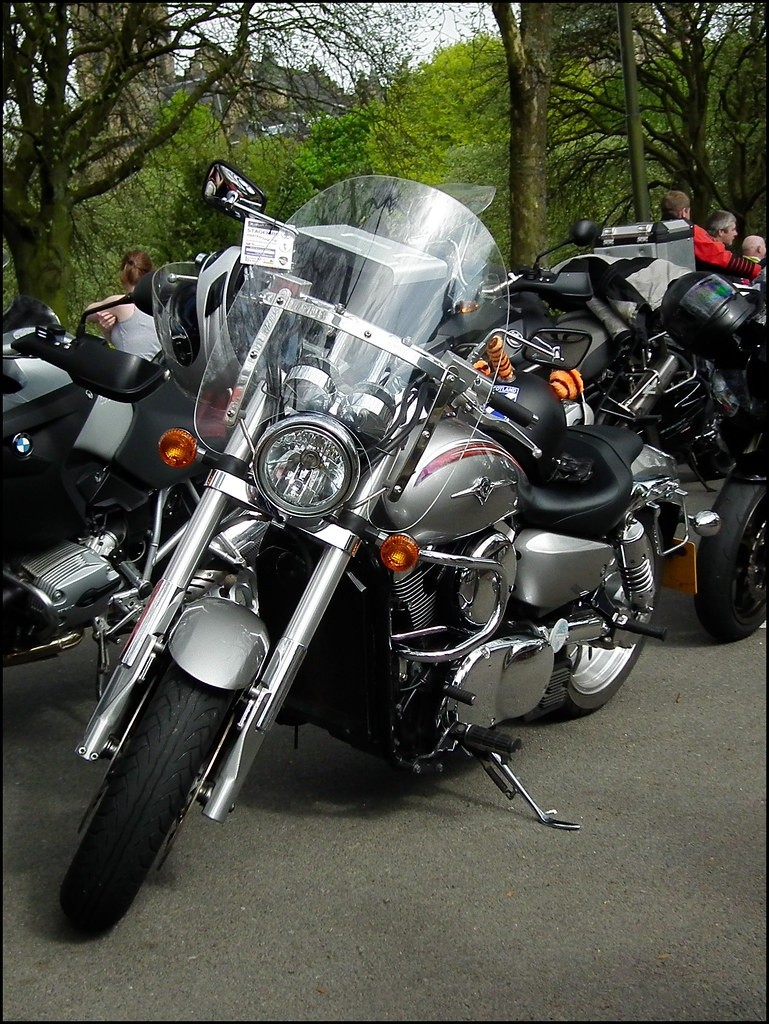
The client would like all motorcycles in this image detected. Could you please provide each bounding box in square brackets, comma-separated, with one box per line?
[61, 160, 721, 919]
[2, 269, 224, 669]
[661, 258, 766, 645]
[508, 216, 697, 423]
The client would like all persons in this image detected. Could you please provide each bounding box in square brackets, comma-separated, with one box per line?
[85, 249, 162, 362]
[742, 235, 766, 263]
[660, 191, 767, 280]
[706, 209, 739, 248]
